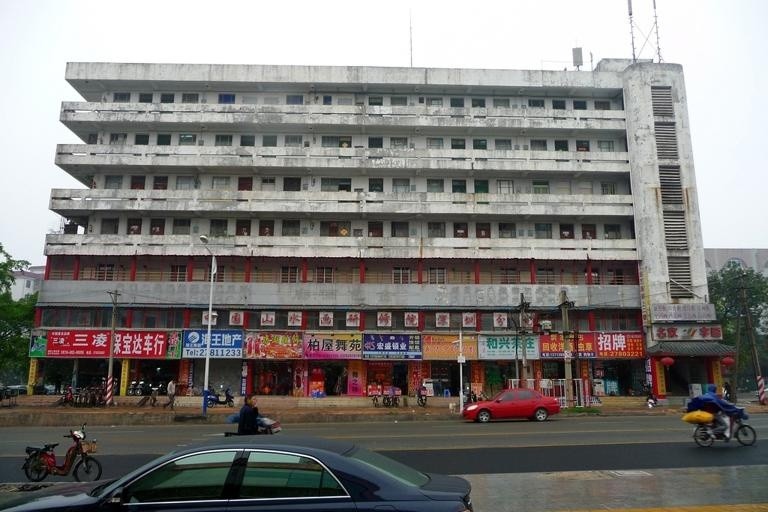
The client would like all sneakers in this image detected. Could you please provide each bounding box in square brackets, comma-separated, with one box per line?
[706, 431, 716, 439]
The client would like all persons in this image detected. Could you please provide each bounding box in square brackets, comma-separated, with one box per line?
[237, 392, 259, 435]
[702, 384, 729, 438]
[162, 376, 177, 410]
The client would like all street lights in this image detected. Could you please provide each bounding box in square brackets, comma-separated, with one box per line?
[199, 235, 215, 415]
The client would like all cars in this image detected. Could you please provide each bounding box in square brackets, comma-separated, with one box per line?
[463, 388, 560, 423]
[1, 435, 473, 512]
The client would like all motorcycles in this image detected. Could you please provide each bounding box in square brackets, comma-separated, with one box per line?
[693, 405, 757, 448]
[207, 385, 234, 407]
[372, 394, 398, 407]
[22, 422, 102, 482]
[127, 378, 166, 396]
[62, 376, 119, 408]
[417, 386, 427, 406]
